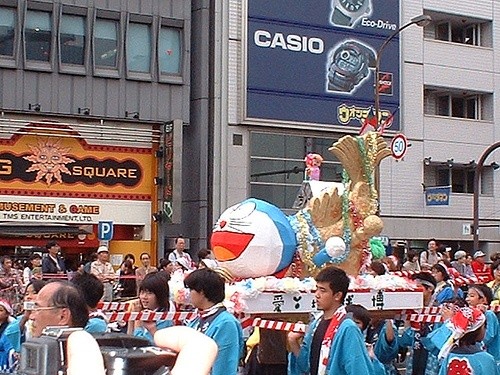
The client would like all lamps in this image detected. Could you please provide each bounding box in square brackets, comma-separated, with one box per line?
[153, 176, 163, 185]
[125, 111, 140, 120]
[483, 162, 500, 170]
[152, 211, 162, 222]
[423, 157, 432, 165]
[78, 107, 90, 116]
[440, 158, 454, 167]
[28, 103, 41, 113]
[154, 149, 163, 159]
[462, 159, 478, 169]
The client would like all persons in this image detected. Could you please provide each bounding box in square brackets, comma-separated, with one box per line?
[186, 268, 244, 375]
[288, 267, 375, 375]
[1, 238, 219, 375]
[347, 239, 500, 375]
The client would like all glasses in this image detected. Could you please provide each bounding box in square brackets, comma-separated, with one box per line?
[29, 304, 55, 314]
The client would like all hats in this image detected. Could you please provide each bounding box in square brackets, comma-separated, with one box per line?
[455, 250, 467, 259]
[97, 246, 109, 254]
[473, 250, 486, 261]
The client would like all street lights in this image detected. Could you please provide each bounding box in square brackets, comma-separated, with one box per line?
[373, 14, 434, 217]
